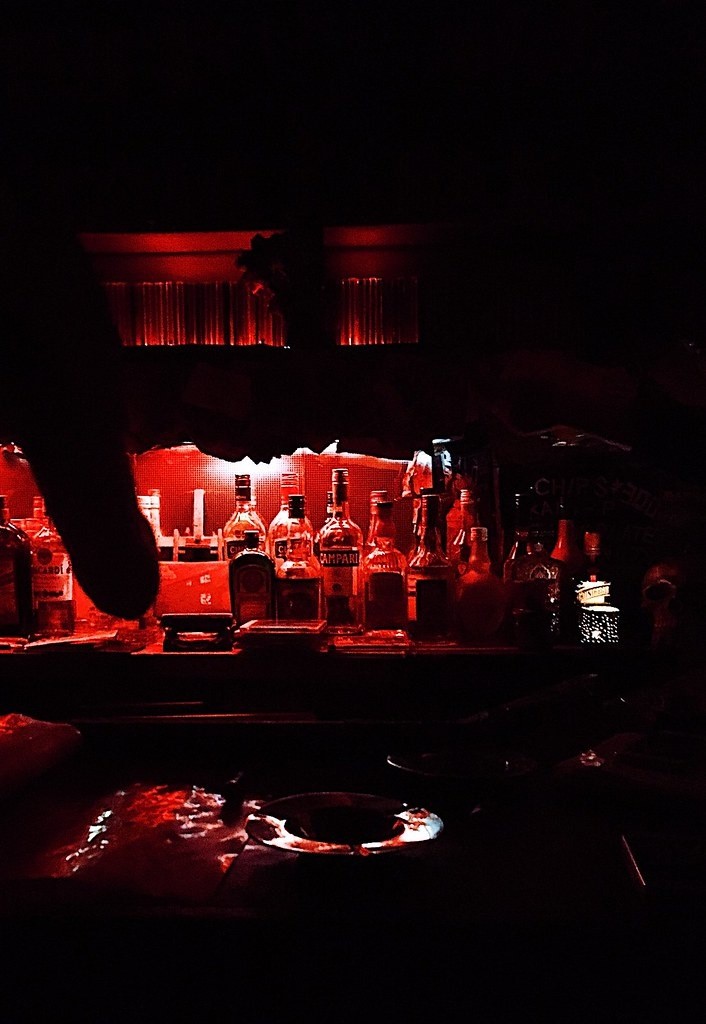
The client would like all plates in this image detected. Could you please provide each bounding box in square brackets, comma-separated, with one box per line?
[243, 791, 445, 856]
[387, 747, 538, 785]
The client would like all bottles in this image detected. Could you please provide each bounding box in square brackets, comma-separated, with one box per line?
[0, 494, 75, 637]
[223, 468, 683, 644]
